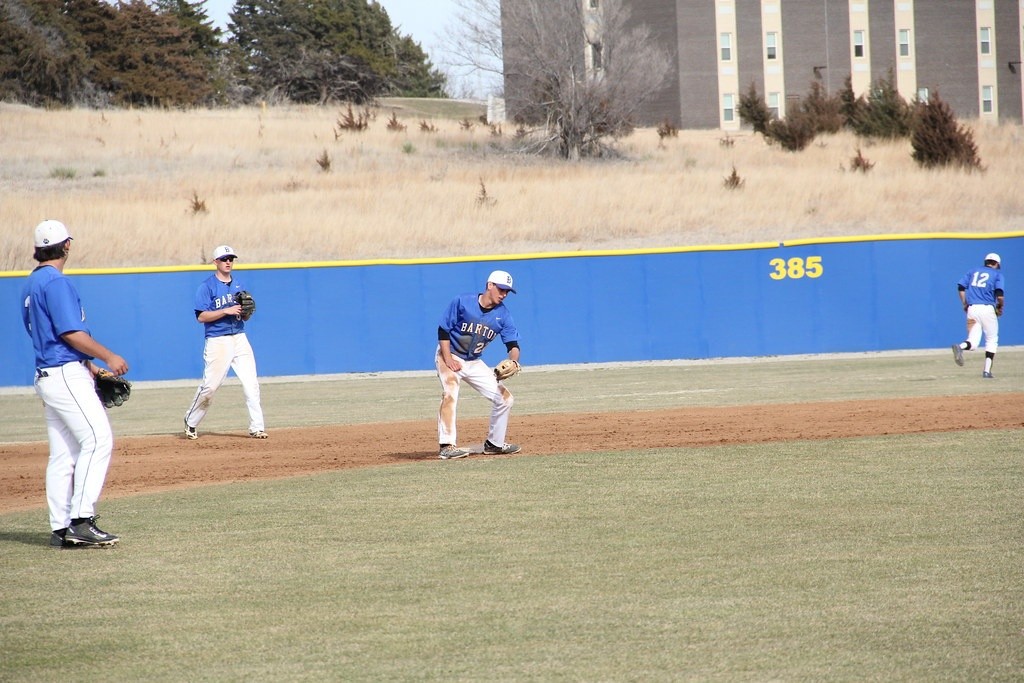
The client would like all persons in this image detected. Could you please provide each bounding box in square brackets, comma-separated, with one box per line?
[182, 245, 270, 441]
[18, 220, 131, 549]
[434, 270, 522, 457]
[952, 251, 1005, 378]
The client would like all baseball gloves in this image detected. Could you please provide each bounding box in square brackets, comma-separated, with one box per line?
[494, 359, 522, 380]
[92, 368, 132, 408]
[995, 304, 1004, 317]
[234, 291, 255, 321]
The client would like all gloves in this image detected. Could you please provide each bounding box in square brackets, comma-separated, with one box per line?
[36, 367, 48, 378]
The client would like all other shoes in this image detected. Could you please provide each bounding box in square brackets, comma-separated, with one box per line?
[952, 344, 964, 366]
[983, 371, 993, 378]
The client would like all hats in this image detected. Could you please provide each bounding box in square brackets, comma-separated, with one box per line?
[985, 253, 1001, 268]
[212, 245, 238, 260]
[488, 270, 517, 294]
[33, 219, 74, 247]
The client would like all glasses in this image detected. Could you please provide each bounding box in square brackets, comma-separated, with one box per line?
[217, 256, 234, 262]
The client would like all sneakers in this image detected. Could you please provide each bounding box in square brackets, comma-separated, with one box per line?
[184, 418, 198, 440]
[49, 531, 69, 549]
[483, 441, 521, 454]
[65, 514, 120, 546]
[437, 445, 469, 459]
[250, 431, 269, 439]
